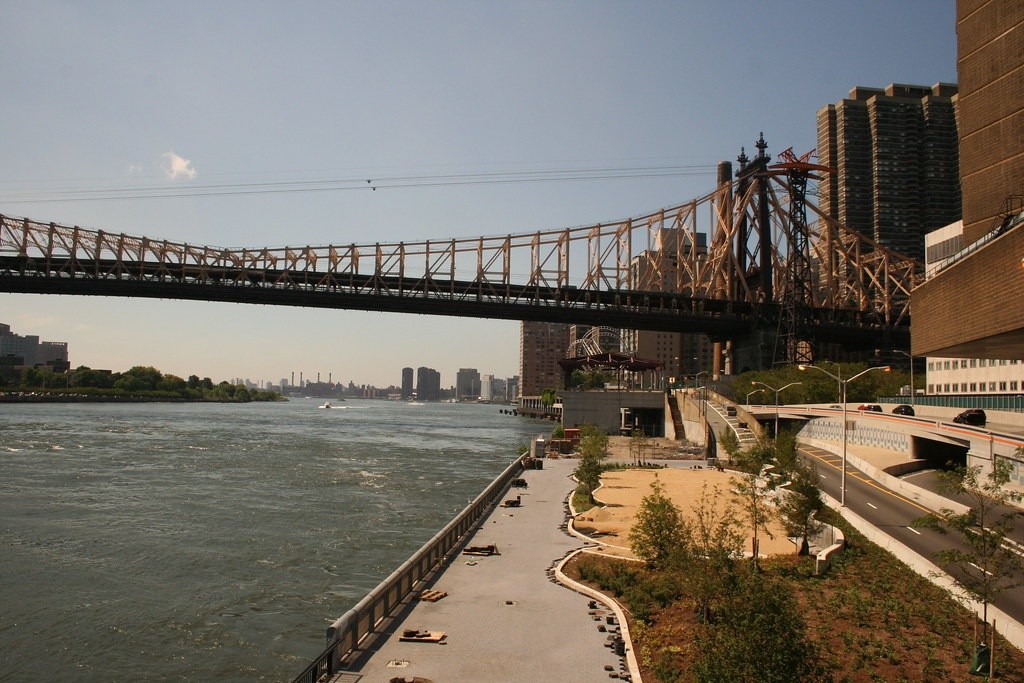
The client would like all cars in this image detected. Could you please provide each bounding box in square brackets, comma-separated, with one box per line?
[867, 404, 882, 412]
[858, 403, 869, 410]
[892, 405, 914, 416]
[953, 409, 986, 426]
[829, 405, 842, 409]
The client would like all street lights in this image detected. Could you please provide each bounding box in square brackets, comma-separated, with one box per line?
[695, 371, 708, 393]
[798, 364, 889, 507]
[893, 350, 914, 407]
[746, 389, 765, 413]
[824, 361, 841, 404]
[751, 380, 803, 444]
